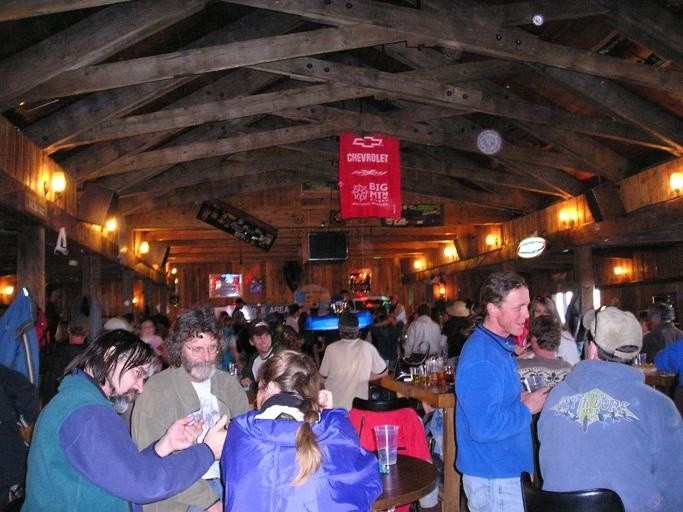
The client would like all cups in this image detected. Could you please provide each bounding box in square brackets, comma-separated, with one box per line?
[233, 367, 241, 381]
[410, 360, 454, 385]
[373, 425, 401, 465]
[637, 353, 647, 365]
[524, 374, 543, 394]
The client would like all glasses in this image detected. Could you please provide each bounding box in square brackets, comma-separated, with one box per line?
[184, 342, 220, 356]
[121, 352, 151, 384]
[592, 305, 607, 342]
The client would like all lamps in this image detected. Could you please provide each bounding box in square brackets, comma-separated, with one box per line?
[51, 171, 66, 194]
[560, 208, 574, 228]
[670, 172, 683, 198]
[485, 234, 499, 251]
[444, 245, 459, 264]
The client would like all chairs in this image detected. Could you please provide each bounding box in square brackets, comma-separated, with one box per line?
[520, 471, 625, 512]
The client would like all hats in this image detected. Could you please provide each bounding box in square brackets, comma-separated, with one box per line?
[446, 300, 470, 317]
[583, 306, 643, 360]
[338, 313, 359, 333]
[248, 320, 274, 335]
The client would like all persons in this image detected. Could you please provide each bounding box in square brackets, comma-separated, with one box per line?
[45, 281, 63, 328]
[137, 319, 164, 357]
[441, 300, 473, 360]
[103, 301, 129, 330]
[340, 289, 353, 309]
[317, 312, 389, 412]
[535, 306, 683, 512]
[18, 327, 229, 512]
[265, 311, 290, 354]
[282, 326, 326, 368]
[231, 298, 256, 341]
[50, 313, 93, 380]
[216, 312, 239, 372]
[453, 270, 552, 512]
[0, 365, 42, 512]
[239, 318, 280, 392]
[285, 303, 304, 333]
[129, 305, 250, 512]
[638, 311, 653, 337]
[298, 305, 304, 315]
[642, 302, 683, 363]
[529, 294, 582, 368]
[514, 314, 574, 396]
[124, 313, 139, 333]
[309, 302, 320, 316]
[653, 336, 683, 417]
[403, 302, 442, 358]
[367, 297, 407, 357]
[218, 348, 384, 512]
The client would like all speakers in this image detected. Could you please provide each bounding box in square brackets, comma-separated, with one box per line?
[308, 233, 348, 262]
[585, 181, 625, 222]
[75, 182, 120, 229]
[146, 240, 170, 268]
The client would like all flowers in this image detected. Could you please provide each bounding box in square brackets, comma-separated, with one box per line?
[551, 272, 570, 287]
